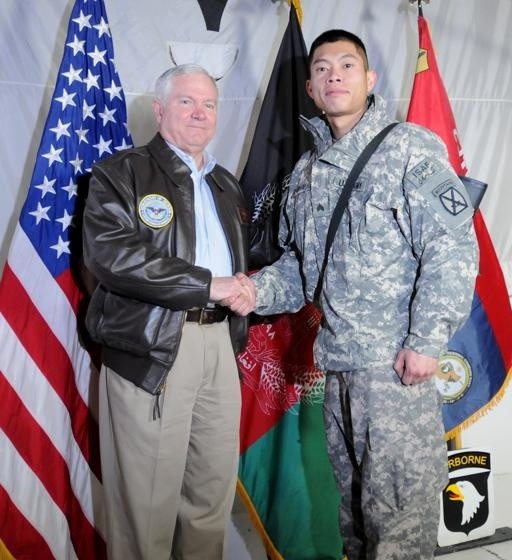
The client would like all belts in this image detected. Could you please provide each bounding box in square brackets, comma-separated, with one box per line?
[186, 308, 230, 325]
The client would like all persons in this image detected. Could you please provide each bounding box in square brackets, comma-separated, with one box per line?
[217, 27, 484, 560]
[82, 64, 252, 560]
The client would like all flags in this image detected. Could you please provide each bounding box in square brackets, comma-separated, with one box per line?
[401, 2, 512, 443]
[2, 0, 133, 560]
[237, 2, 345, 560]
